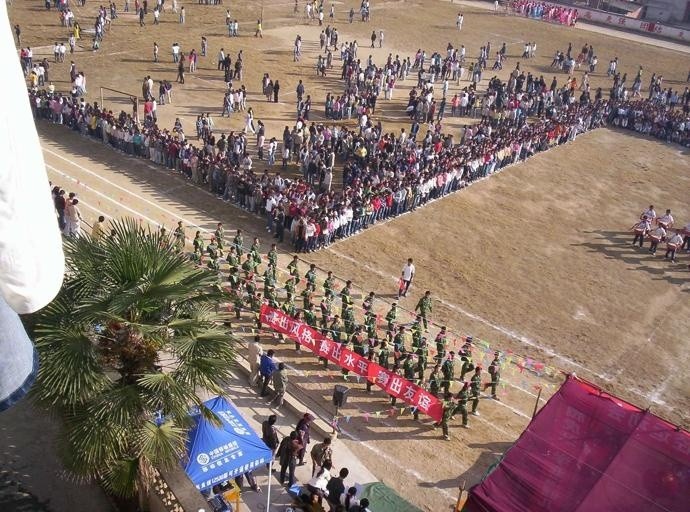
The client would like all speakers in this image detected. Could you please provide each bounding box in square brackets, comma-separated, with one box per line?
[333, 385, 349, 406]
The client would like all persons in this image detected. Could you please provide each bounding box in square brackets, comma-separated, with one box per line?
[262, 412, 315, 488]
[291, 459, 369, 512]
[6, 0, 690, 273]
[310, 437, 333, 479]
[169, 274, 503, 440]
[235, 470, 262, 493]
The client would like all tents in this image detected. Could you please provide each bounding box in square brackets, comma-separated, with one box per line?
[155, 396, 274, 512]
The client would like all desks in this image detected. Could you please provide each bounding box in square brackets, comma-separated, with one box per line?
[220, 478, 242, 512]
